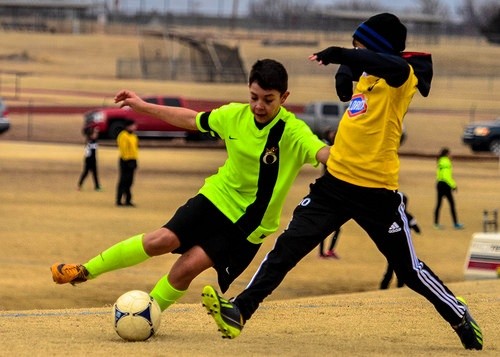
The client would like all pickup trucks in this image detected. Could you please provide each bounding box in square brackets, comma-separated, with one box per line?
[293, 100, 406, 149]
[82, 94, 224, 146]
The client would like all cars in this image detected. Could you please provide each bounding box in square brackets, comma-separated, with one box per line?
[461, 118, 500, 156]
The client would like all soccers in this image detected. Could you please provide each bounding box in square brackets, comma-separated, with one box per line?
[111, 289, 162, 342]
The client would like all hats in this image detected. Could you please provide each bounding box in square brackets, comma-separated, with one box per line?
[352, 12, 407, 54]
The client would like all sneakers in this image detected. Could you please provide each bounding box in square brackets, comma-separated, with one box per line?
[453, 296, 485, 350]
[49, 262, 87, 287]
[201, 285, 245, 339]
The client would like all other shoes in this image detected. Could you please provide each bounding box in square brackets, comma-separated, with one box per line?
[116, 201, 124, 206]
[454, 224, 463, 229]
[317, 253, 330, 260]
[325, 250, 341, 259]
[125, 202, 134, 206]
[434, 224, 443, 228]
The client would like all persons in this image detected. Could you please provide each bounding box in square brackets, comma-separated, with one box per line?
[78, 121, 104, 191]
[317, 128, 337, 178]
[319, 226, 340, 257]
[377, 192, 421, 290]
[200, 12, 483, 350]
[48, 59, 333, 320]
[433, 146, 464, 228]
[115, 118, 139, 207]
